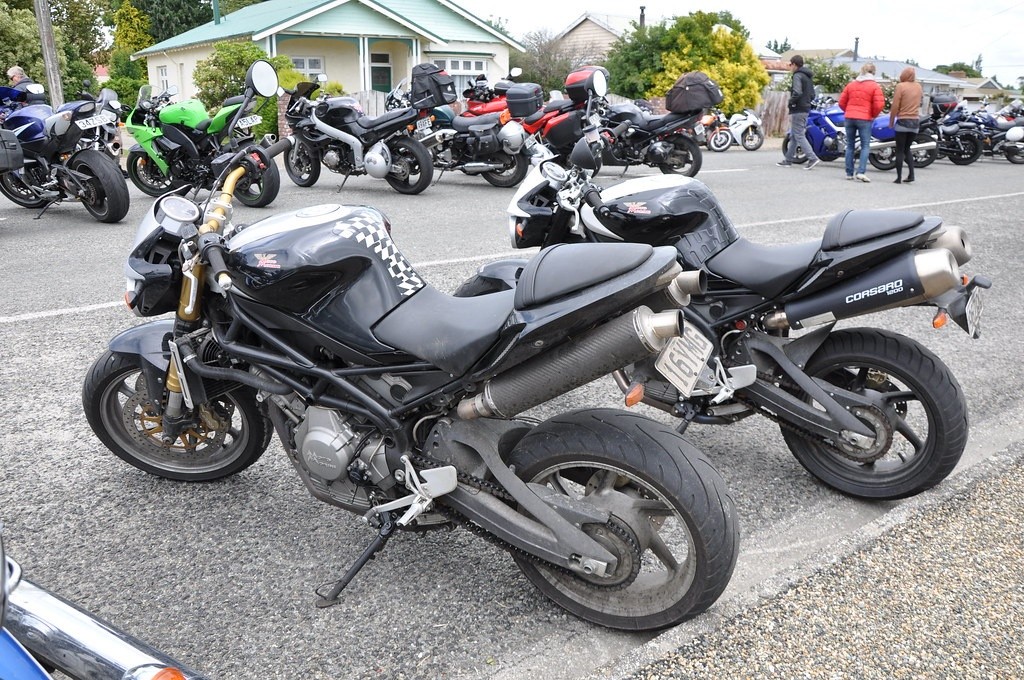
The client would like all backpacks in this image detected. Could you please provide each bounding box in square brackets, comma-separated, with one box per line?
[665, 71, 723, 113]
[410, 63, 457, 108]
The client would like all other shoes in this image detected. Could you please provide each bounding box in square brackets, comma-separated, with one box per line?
[846, 176, 853, 179]
[857, 174, 870, 182]
[903, 178, 914, 182]
[893, 179, 901, 184]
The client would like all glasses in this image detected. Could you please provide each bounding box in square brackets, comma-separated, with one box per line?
[10, 75, 15, 81]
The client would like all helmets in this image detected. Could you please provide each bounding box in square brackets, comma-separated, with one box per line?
[497, 120, 525, 155]
[364, 143, 392, 178]
[1006, 126, 1024, 141]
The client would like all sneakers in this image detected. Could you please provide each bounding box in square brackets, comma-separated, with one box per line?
[804, 158, 820, 169]
[776, 160, 791, 167]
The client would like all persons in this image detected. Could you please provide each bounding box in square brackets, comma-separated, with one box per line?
[7, 66, 47, 106]
[776, 54, 821, 170]
[839, 63, 885, 181]
[889, 67, 923, 184]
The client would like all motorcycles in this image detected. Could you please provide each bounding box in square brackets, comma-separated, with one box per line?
[452, 68, 993, 501]
[125, 85, 280, 208]
[277, 72, 448, 196]
[586, 85, 709, 177]
[659, 107, 765, 153]
[782, 85, 1024, 171]
[385, 76, 543, 188]
[81, 57, 759, 633]
[1, 77, 131, 224]
[460, 66, 607, 178]
